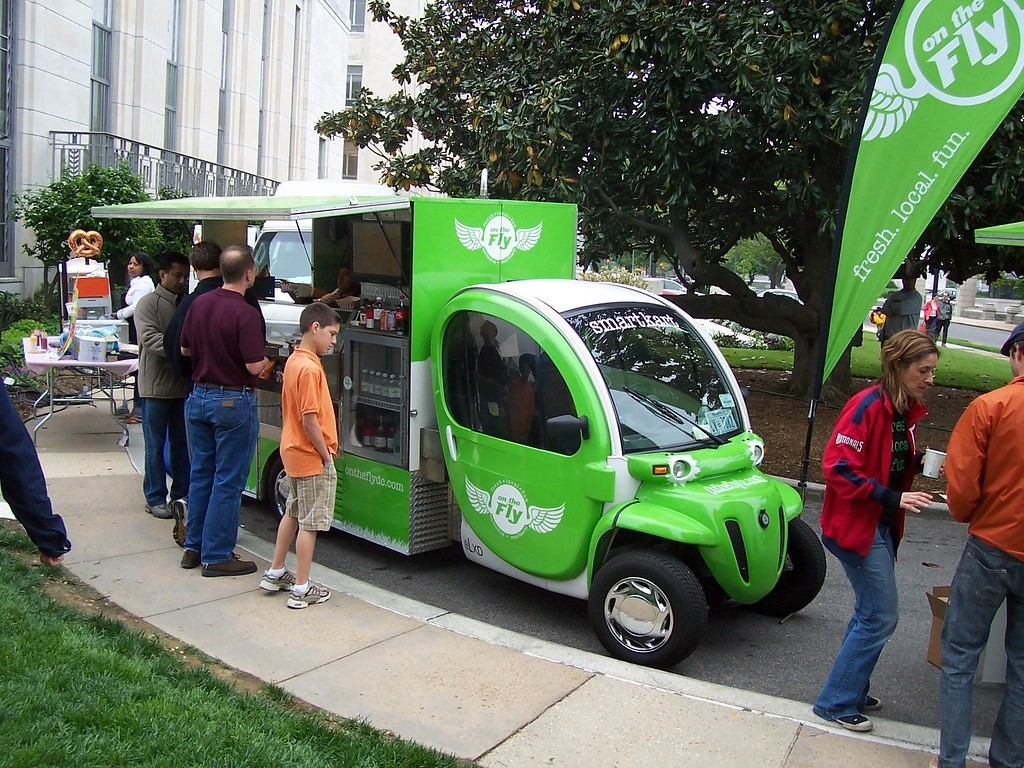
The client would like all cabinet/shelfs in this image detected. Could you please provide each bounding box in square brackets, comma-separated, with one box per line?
[343, 326, 409, 470]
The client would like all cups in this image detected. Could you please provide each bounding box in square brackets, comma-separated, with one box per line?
[922, 449, 947, 479]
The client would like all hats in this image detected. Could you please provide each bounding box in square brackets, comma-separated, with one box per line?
[1000, 320, 1024, 357]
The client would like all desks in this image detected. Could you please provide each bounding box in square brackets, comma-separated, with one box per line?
[22, 337, 139, 447]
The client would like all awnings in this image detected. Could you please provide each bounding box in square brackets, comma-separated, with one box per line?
[91, 195, 410, 272]
[975, 221, 1024, 246]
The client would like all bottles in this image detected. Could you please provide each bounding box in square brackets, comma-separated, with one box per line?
[359, 367, 406, 398]
[65, 297, 114, 319]
[362, 409, 400, 453]
[698, 397, 711, 425]
[360, 295, 408, 335]
[116, 430, 128, 448]
[257, 399, 282, 428]
[279, 343, 289, 356]
[29, 327, 47, 354]
[82, 384, 88, 397]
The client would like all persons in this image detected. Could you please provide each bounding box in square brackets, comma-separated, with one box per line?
[133, 241, 268, 577]
[111, 254, 155, 424]
[279, 262, 366, 302]
[260, 303, 341, 609]
[813, 329, 947, 732]
[929, 321, 1024, 768]
[0, 377, 72, 568]
[923, 292, 952, 345]
[870, 277, 922, 351]
[473, 321, 534, 438]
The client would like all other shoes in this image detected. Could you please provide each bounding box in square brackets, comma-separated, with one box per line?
[929, 755, 939, 768]
[118, 414, 142, 424]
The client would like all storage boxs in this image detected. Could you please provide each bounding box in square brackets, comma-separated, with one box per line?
[74, 336, 106, 362]
[925, 586, 953, 668]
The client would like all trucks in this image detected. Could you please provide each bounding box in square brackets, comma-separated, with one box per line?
[189, 179, 414, 354]
[90, 197, 826, 671]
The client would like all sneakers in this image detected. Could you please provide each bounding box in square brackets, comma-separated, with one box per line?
[286, 582, 331, 609]
[144, 500, 173, 518]
[260, 567, 297, 591]
[171, 499, 189, 547]
[833, 713, 873, 732]
[181, 547, 202, 569]
[858, 695, 881, 711]
[201, 552, 258, 577]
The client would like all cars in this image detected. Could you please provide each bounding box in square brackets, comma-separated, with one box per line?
[643, 277, 806, 305]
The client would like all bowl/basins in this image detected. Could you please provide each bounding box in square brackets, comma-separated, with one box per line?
[336, 295, 359, 309]
